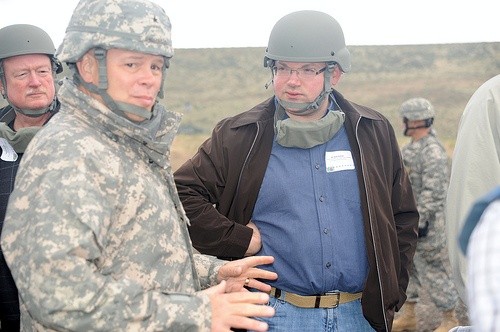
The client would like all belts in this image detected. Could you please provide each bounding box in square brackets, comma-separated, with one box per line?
[268, 287, 363, 309]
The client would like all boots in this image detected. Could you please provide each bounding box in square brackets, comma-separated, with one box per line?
[433, 308, 459, 332]
[391, 303, 418, 332]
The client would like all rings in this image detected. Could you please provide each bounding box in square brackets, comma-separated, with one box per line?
[244, 279, 250, 287]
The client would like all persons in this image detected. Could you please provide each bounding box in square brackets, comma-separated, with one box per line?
[391, 98, 470, 332]
[0, 0, 277, 332]
[173, 10, 419, 332]
[0, 23, 61, 332]
[447, 74, 500, 332]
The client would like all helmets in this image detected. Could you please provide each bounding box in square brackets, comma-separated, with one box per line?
[263, 10, 351, 73]
[0, 24, 56, 59]
[53, 0, 174, 64]
[400, 98, 435, 121]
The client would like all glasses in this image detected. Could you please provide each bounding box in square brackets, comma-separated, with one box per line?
[271, 60, 326, 78]
